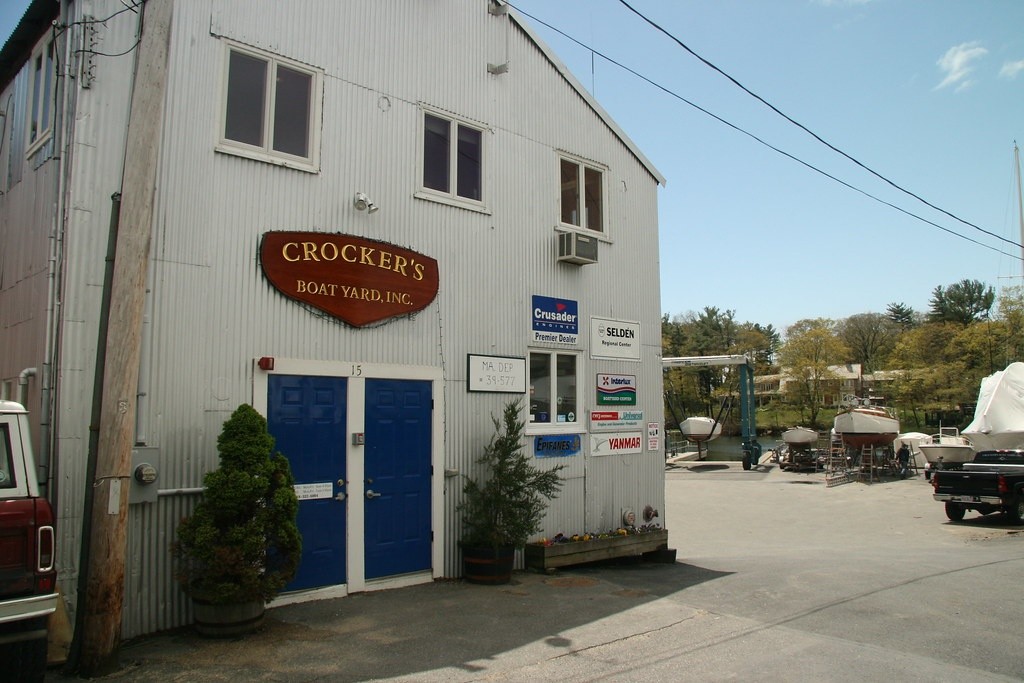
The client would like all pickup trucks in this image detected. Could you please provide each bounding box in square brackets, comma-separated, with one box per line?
[933, 449, 1024, 529]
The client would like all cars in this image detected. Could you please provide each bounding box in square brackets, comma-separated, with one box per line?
[839, 394, 870, 408]
[0, 398, 60, 625]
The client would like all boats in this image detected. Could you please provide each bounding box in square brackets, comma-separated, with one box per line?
[781, 426, 819, 446]
[679, 415, 722, 442]
[918, 419, 978, 470]
[834, 394, 900, 453]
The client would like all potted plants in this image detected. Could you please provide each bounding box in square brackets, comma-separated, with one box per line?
[450, 395, 569, 585]
[166, 403, 301, 638]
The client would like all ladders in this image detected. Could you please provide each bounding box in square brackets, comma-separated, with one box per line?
[901, 440, 918, 476]
[815, 431, 830, 477]
[856, 442, 881, 484]
[825, 432, 848, 477]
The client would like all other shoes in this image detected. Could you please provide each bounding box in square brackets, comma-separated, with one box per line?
[901, 474, 905, 480]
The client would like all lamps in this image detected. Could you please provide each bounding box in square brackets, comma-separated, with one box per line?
[356, 192, 378, 214]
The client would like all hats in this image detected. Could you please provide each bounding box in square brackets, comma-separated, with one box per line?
[901, 441, 908, 447]
